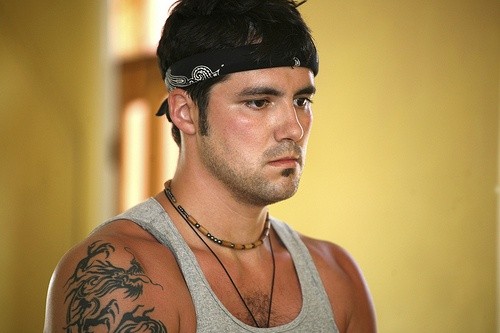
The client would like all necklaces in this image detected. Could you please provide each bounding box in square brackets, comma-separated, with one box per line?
[164, 180, 270, 251]
[164, 190, 278, 327]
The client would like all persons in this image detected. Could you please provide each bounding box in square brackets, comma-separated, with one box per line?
[43, 1, 377, 332]
